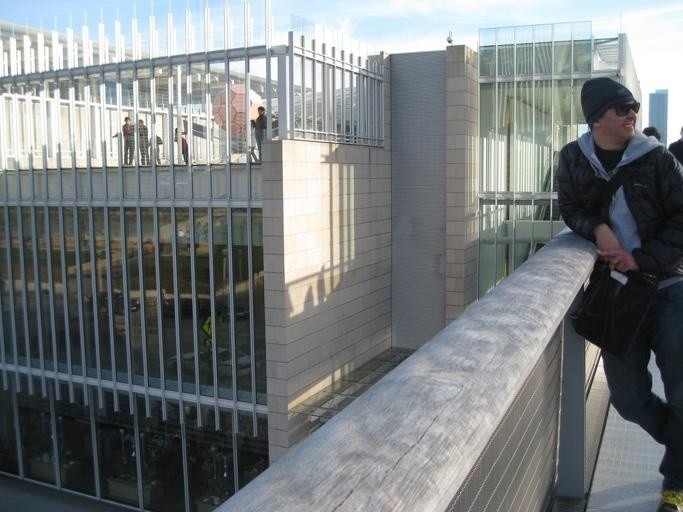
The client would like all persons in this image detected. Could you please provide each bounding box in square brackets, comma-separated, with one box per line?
[642, 126, 683, 165]
[148, 135, 164, 164]
[122, 116, 135, 165]
[557, 78, 683, 512]
[174, 128, 188, 164]
[138, 119, 149, 165]
[251, 106, 268, 162]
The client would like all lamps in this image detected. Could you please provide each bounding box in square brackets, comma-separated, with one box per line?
[447, 29, 453, 46]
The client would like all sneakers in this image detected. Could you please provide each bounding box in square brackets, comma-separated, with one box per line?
[658, 492, 682, 511]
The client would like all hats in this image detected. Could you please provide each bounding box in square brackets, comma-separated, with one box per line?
[581, 77, 640, 125]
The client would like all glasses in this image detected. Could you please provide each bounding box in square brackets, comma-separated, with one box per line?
[608, 102, 640, 117]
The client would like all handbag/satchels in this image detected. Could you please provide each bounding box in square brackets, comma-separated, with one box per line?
[571, 260, 657, 363]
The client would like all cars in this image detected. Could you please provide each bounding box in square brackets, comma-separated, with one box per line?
[168, 345, 259, 378]
[90, 292, 141, 315]
[126, 237, 166, 258]
[201, 303, 250, 323]
